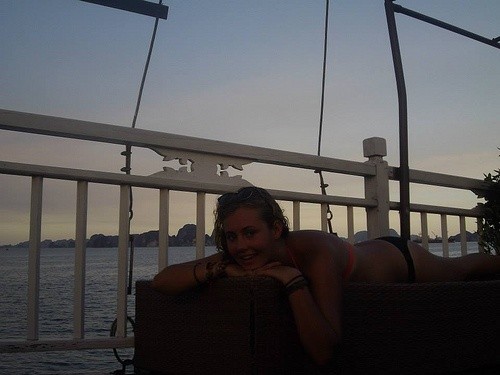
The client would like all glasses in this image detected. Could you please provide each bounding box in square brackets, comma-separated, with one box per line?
[218, 187, 259, 207]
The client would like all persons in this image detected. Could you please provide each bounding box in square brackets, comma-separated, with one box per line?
[143, 184, 500, 367]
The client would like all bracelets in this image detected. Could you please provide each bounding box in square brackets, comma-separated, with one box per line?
[192, 262, 204, 287]
[205, 259, 219, 280]
[216, 259, 233, 280]
[284, 273, 306, 287]
[210, 259, 223, 280]
[283, 277, 308, 294]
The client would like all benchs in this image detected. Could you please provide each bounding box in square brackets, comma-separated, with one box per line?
[134, 279, 500, 375]
